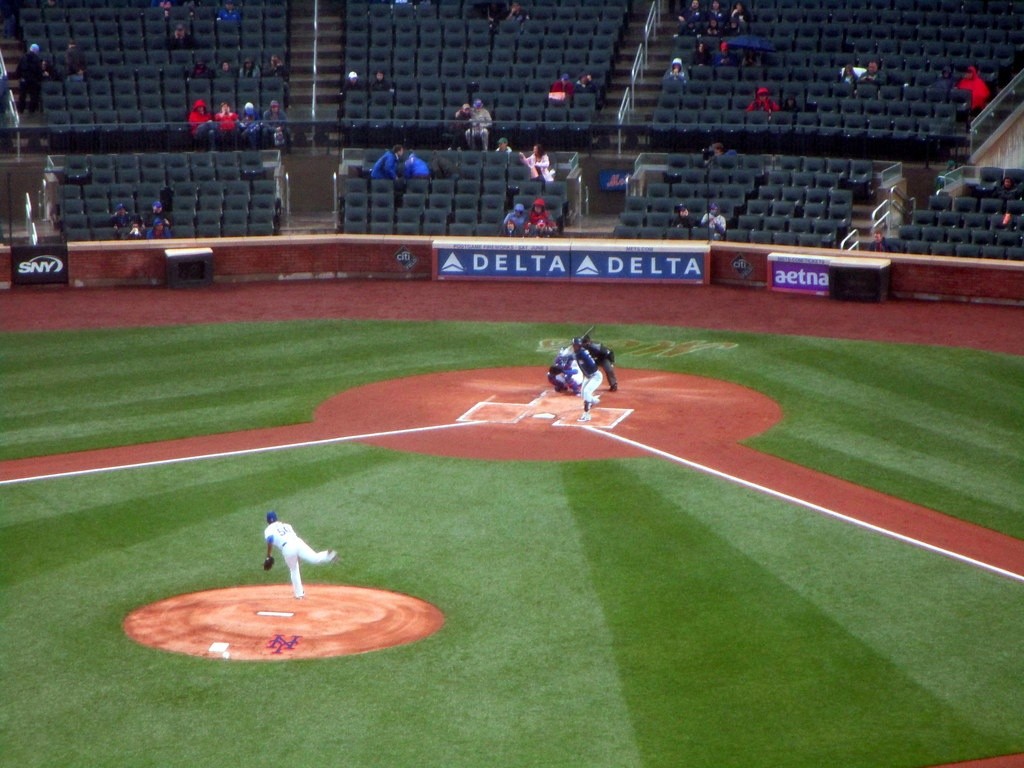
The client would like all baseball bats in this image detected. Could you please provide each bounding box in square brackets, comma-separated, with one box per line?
[581, 325, 595, 339]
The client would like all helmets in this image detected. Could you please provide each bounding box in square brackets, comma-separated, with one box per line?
[570, 337, 582, 345]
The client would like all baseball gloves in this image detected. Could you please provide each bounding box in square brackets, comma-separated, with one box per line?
[263, 556, 275, 571]
[550, 365, 561, 376]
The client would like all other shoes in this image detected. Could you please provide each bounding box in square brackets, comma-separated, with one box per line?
[610, 383, 618, 391]
[577, 412, 591, 422]
[296, 595, 304, 600]
[328, 549, 338, 561]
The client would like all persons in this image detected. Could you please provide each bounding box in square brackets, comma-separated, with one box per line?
[264, 512, 338, 599]
[572, 337, 603, 423]
[0, 0, 1023, 252]
[548, 335, 618, 397]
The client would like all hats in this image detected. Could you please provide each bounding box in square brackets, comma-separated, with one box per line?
[267, 512, 277, 524]
[115, 202, 124, 210]
[712, 143, 724, 149]
[583, 335, 590, 341]
[271, 100, 281, 108]
[153, 217, 163, 226]
[946, 160, 956, 169]
[677, 203, 687, 211]
[245, 102, 254, 114]
[549, 363, 562, 375]
[152, 201, 163, 208]
[562, 73, 570, 80]
[349, 71, 358, 79]
[474, 99, 482, 107]
[496, 137, 509, 144]
[462, 103, 471, 110]
[709, 203, 719, 210]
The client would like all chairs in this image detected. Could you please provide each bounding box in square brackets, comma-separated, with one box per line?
[15, 1, 1024, 261]
